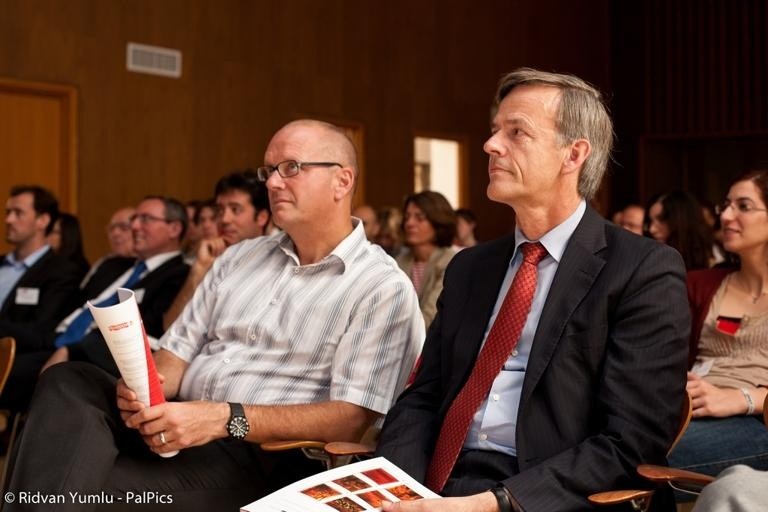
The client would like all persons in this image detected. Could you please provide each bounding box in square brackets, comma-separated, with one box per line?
[0, 67, 767, 510]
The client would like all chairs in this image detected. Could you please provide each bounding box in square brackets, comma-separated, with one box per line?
[0, 333, 722, 509]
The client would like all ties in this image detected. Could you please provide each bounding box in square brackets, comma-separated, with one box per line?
[54, 260, 145, 348]
[423, 242, 547, 495]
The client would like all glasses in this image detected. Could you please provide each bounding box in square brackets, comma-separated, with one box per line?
[257, 160, 342, 182]
[715, 203, 768, 215]
